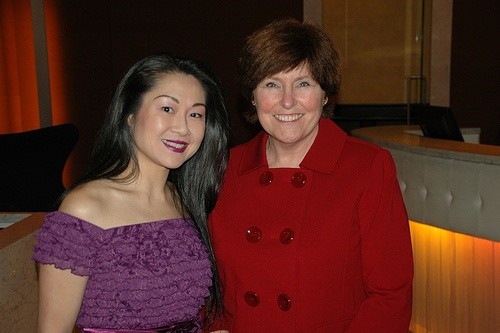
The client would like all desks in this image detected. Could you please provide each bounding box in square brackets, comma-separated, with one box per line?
[0, 210, 81, 333]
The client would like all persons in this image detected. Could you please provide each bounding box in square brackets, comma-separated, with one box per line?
[31, 53, 231, 333]
[201, 19, 414, 333]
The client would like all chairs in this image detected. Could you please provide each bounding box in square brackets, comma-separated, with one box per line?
[0, 123, 79, 212]
